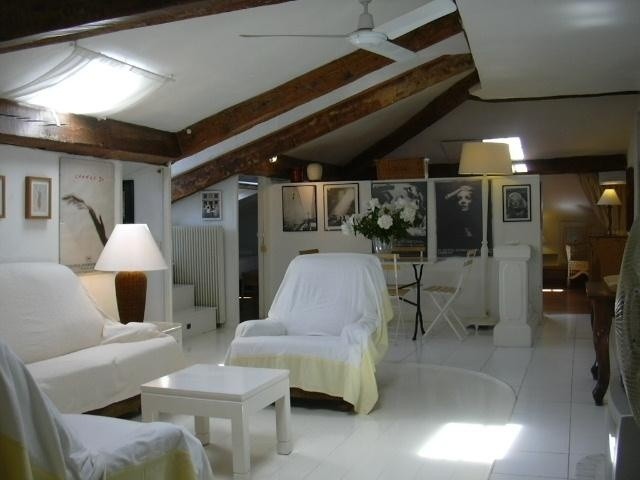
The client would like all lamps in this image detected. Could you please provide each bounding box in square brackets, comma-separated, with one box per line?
[93, 224, 169, 324]
[0, 44, 171, 120]
[596, 188, 623, 235]
[458, 141, 513, 331]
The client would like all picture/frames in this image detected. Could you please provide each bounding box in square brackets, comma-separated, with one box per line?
[0, 175, 6, 219]
[322, 182, 360, 232]
[25, 176, 52, 220]
[58, 156, 116, 274]
[200, 191, 222, 221]
[434, 179, 493, 258]
[282, 185, 318, 232]
[501, 184, 532, 223]
[370, 181, 428, 259]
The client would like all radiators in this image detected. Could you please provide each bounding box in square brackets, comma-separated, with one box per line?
[172, 225, 225, 328]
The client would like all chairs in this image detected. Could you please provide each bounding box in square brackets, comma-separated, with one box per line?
[374, 254, 410, 337]
[565, 244, 592, 288]
[421, 248, 477, 340]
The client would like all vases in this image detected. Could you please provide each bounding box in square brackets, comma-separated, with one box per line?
[372, 237, 392, 260]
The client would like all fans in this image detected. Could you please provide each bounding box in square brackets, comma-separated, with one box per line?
[239, 0, 457, 65]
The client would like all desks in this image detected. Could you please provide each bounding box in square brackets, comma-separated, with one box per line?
[381, 257, 435, 340]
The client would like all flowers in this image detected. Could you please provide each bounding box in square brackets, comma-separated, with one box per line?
[341, 197, 421, 245]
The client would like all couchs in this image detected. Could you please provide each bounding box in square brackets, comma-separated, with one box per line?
[223, 253, 395, 414]
[0, 261, 184, 418]
[0, 336, 217, 480]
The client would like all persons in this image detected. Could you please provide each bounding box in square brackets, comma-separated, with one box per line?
[439, 184, 480, 249]
[507, 192, 527, 218]
